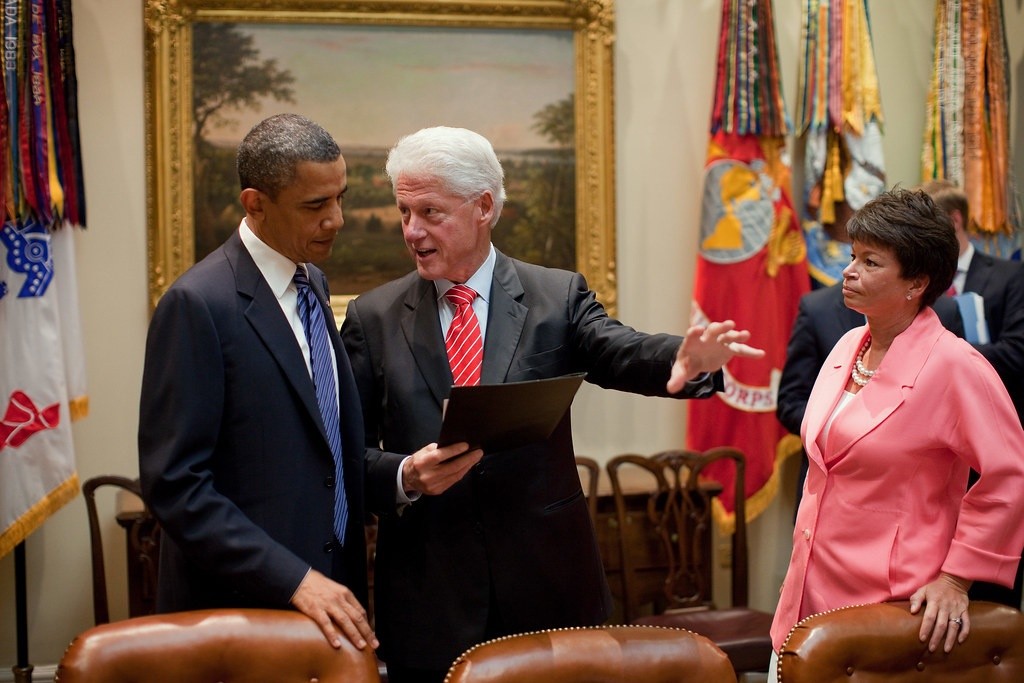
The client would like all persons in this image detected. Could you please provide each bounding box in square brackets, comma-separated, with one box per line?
[774, 274, 966, 528]
[908, 178, 1024, 429]
[137, 113, 380, 652]
[339, 127, 765, 683]
[766, 183, 1024, 683]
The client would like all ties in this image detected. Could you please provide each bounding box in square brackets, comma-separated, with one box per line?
[948, 284, 955, 297]
[442, 283, 484, 387]
[292, 263, 350, 547]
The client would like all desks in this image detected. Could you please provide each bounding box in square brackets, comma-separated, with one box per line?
[117, 463, 725, 630]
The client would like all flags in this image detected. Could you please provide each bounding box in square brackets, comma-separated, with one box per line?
[686, 0, 812, 541]
[0, 0, 90, 555]
[919, 1, 1021, 261]
[792, 0, 889, 292]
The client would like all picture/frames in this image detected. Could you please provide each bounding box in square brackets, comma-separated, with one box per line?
[140, 0, 620, 332]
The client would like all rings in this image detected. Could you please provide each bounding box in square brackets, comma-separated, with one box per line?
[950, 617, 962, 625]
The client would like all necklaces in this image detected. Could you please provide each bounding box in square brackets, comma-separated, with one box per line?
[851, 337, 877, 387]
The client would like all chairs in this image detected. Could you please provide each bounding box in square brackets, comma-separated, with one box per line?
[606, 447, 775, 682]
[777, 601, 1024, 682]
[83, 473, 166, 627]
[54, 607, 380, 682]
[446, 625, 740, 683]
